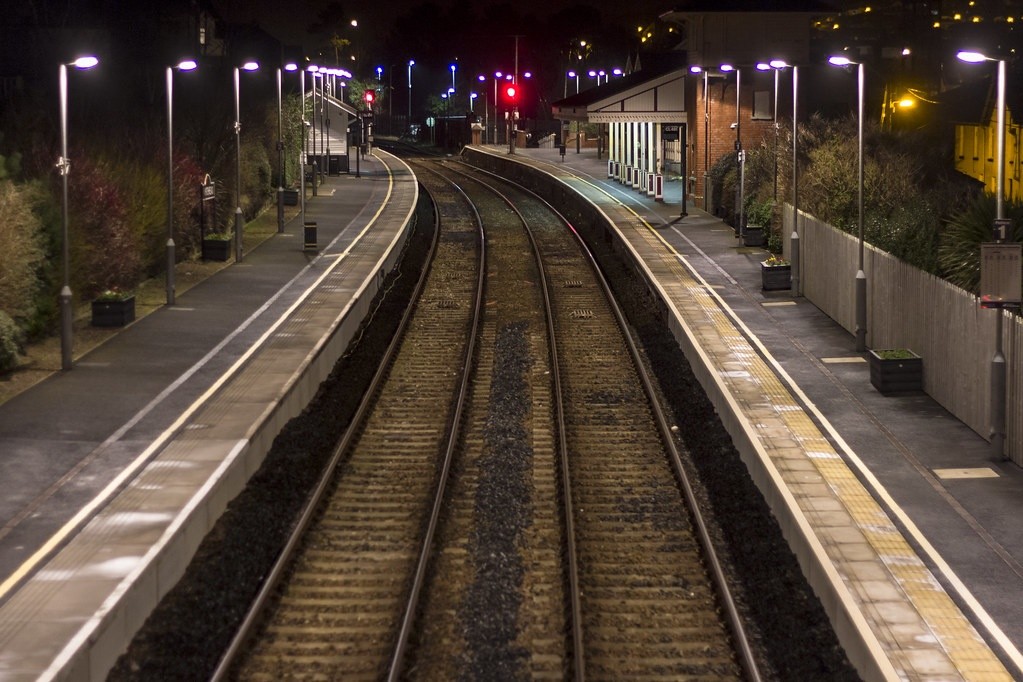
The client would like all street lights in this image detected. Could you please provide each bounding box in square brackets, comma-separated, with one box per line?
[59, 55, 100, 372]
[691, 66, 709, 212]
[957, 49, 1009, 464]
[234, 61, 260, 264]
[276, 62, 299, 234]
[494, 71, 532, 145]
[568, 68, 625, 160]
[302, 65, 353, 228]
[756, 61, 780, 201]
[479, 76, 489, 144]
[770, 58, 801, 299]
[408, 60, 415, 117]
[165, 59, 197, 305]
[441, 65, 478, 112]
[720, 64, 742, 239]
[376, 66, 382, 114]
[828, 54, 868, 351]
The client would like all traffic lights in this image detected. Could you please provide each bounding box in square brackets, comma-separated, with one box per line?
[506, 85, 517, 104]
[365, 89, 375, 103]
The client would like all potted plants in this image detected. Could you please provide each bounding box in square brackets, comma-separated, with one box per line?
[869, 348, 923, 398]
[203, 231, 232, 261]
[743, 223, 767, 247]
[283, 186, 300, 206]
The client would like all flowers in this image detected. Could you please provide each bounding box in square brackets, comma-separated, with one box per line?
[92, 286, 128, 301]
[765, 255, 790, 265]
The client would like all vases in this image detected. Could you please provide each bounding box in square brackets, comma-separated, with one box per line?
[92, 295, 137, 328]
[761, 262, 791, 292]
